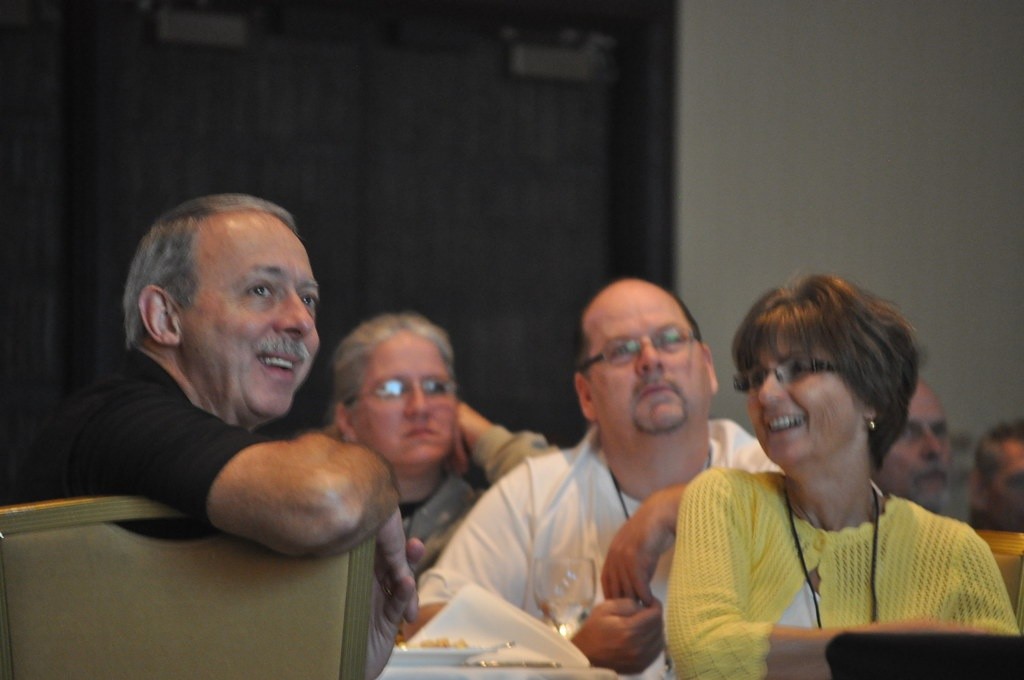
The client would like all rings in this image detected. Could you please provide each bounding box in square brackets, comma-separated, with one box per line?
[381, 581, 393, 599]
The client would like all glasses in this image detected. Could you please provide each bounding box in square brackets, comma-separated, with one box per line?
[733, 352, 838, 392]
[579, 324, 702, 372]
[344, 377, 457, 406]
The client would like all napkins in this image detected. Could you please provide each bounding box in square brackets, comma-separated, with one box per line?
[405, 584, 591, 670]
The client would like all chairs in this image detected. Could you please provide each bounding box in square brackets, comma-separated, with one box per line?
[0, 494, 376, 680]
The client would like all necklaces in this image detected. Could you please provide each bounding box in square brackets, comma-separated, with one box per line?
[608, 449, 712, 521]
[399, 502, 414, 540]
[783, 477, 879, 630]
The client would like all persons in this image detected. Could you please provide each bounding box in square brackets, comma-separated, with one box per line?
[402, 277, 781, 680]
[307, 311, 563, 582]
[13, 192, 425, 680]
[665, 276, 1024, 680]
